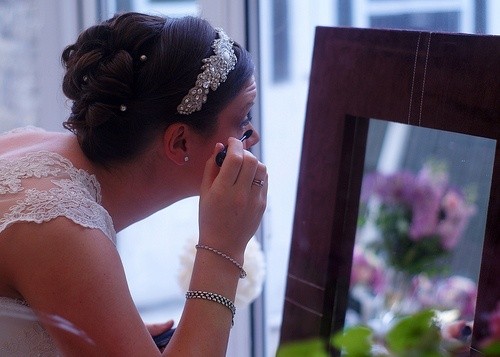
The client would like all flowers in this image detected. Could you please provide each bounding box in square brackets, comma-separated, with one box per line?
[364, 172, 479, 280]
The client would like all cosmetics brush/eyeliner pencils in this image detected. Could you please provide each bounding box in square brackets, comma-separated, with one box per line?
[215, 129, 254, 166]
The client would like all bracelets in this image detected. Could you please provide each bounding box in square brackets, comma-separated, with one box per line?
[195, 244, 249, 280]
[184, 290, 237, 327]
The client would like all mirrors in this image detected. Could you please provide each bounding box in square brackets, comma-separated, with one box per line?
[279, 25, 500, 357]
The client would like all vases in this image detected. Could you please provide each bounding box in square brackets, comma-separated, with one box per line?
[380, 268, 410, 325]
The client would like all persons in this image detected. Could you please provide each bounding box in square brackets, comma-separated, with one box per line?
[0, 12, 272, 357]
[439, 322, 473, 357]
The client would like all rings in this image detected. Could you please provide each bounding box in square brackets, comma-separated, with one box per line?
[252, 179, 265, 189]
